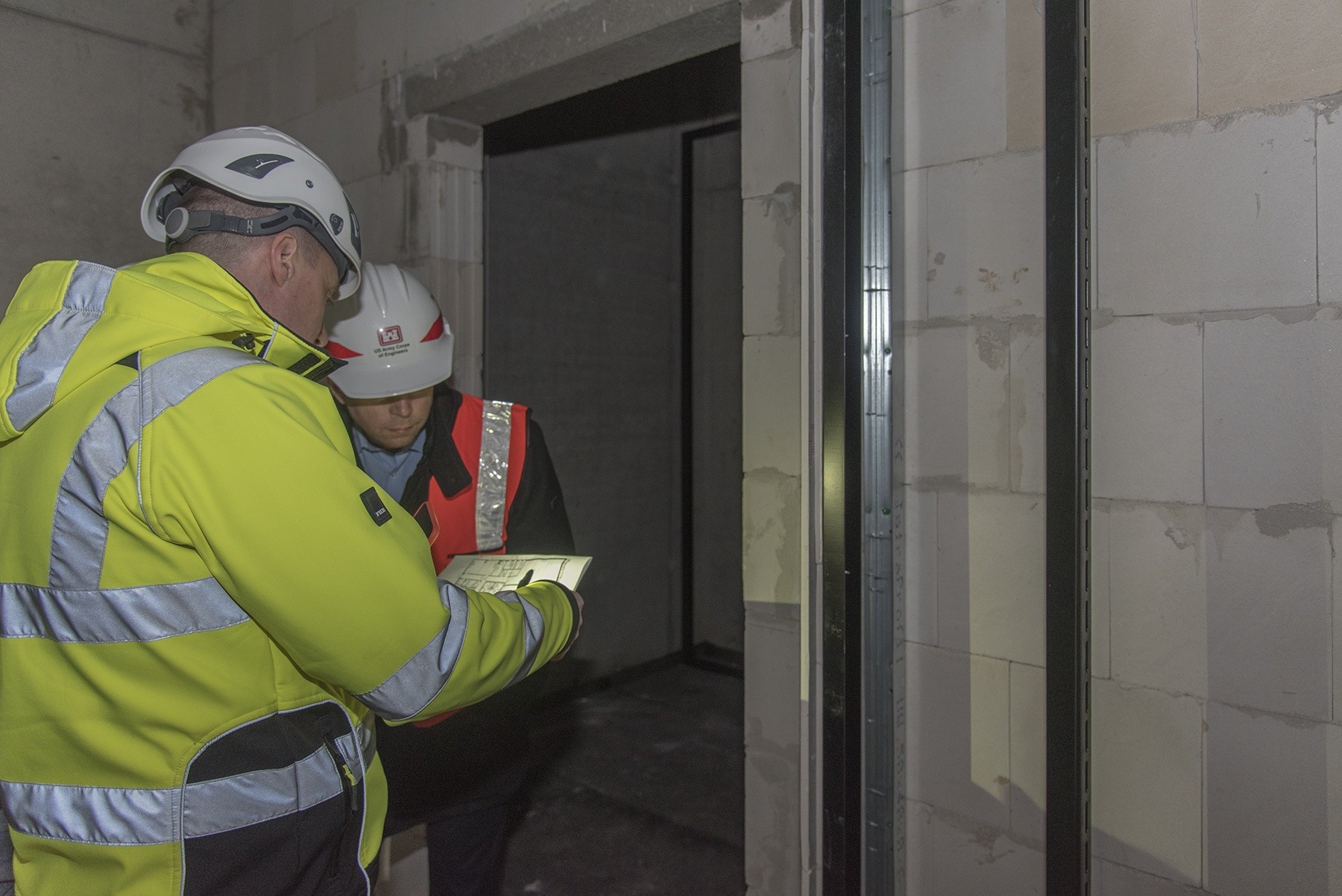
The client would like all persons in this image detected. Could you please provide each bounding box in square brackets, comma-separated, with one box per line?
[0, 123, 586, 896]
[320, 261, 577, 896]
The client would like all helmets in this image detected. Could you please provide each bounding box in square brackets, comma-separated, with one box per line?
[323, 260, 454, 399]
[142, 125, 362, 302]
